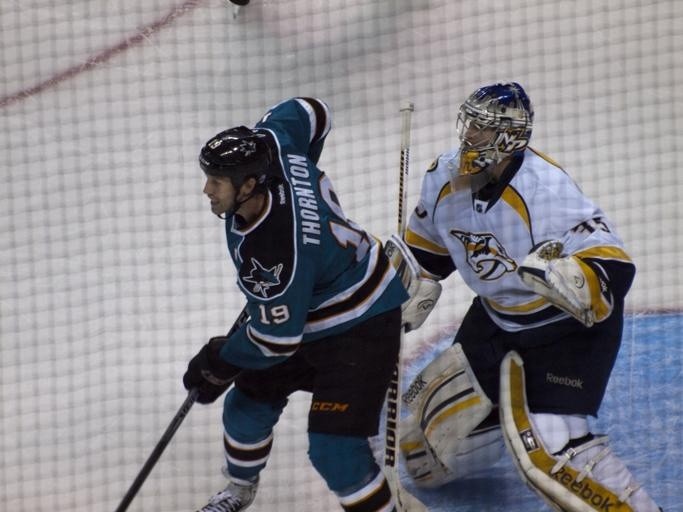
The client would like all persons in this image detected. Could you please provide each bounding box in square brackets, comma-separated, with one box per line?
[181, 95, 410, 511]
[384, 80, 662, 512]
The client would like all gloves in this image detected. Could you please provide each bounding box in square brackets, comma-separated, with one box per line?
[183, 336, 236, 404]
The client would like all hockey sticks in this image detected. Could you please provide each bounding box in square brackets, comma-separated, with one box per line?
[383, 101, 430, 511]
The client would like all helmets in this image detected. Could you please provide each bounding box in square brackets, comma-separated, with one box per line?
[456, 81, 534, 176]
[198, 126, 270, 193]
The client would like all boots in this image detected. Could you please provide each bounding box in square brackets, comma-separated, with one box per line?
[194, 464, 260, 512]
